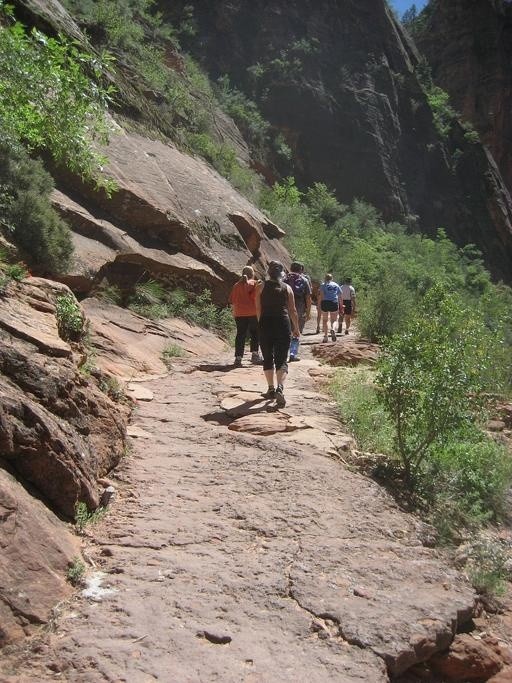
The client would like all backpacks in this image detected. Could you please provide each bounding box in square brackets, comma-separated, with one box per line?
[283, 272, 303, 298]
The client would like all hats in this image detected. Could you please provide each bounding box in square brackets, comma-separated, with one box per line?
[268, 260, 285, 277]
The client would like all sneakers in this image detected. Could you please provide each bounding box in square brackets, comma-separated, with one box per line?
[263, 383, 286, 405]
[234, 357, 242, 368]
[317, 327, 349, 343]
[290, 356, 301, 361]
[252, 352, 261, 364]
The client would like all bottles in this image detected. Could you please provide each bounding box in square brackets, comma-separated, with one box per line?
[288, 335, 300, 358]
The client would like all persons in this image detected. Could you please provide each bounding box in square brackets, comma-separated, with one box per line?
[254, 259, 301, 406]
[228, 259, 357, 368]
